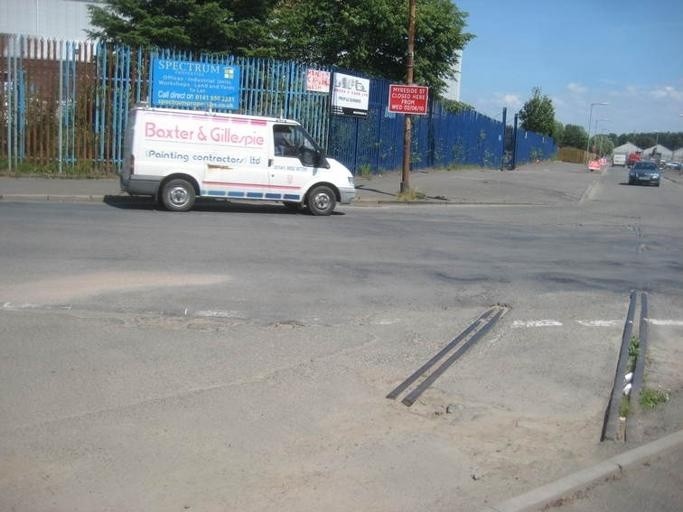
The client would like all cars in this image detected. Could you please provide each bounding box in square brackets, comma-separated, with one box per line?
[662, 161, 683, 170]
[627, 161, 661, 186]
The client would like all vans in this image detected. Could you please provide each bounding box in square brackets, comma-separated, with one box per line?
[120, 106, 356, 216]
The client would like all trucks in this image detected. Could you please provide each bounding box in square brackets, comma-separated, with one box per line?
[613, 154, 627, 168]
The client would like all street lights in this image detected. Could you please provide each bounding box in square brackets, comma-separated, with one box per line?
[586, 101, 610, 161]
